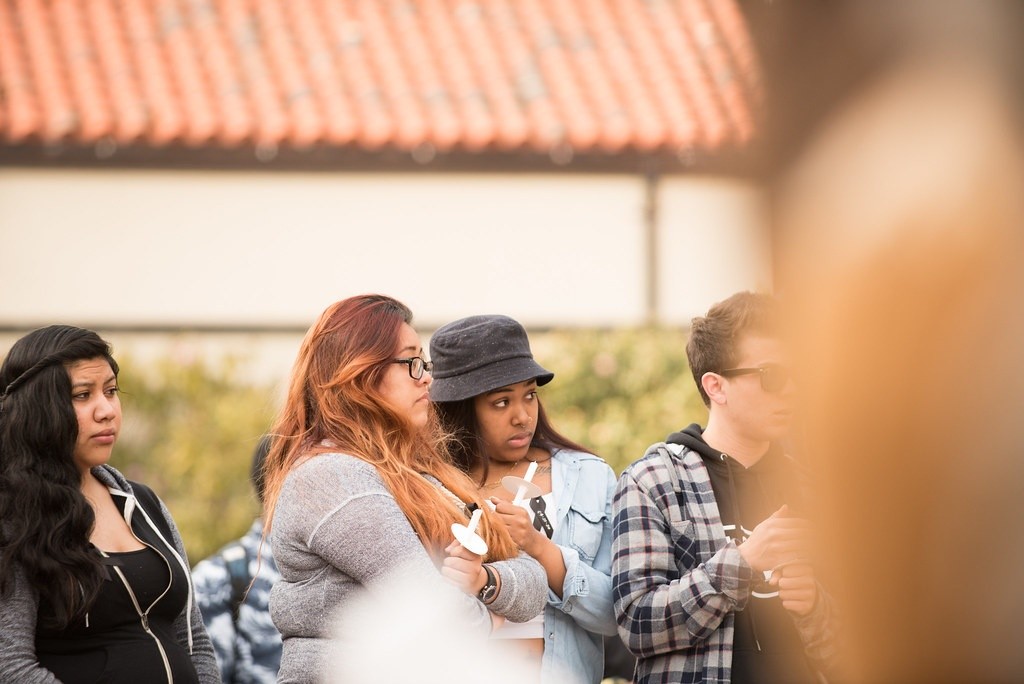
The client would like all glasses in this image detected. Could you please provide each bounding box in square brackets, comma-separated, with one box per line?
[725, 364, 788, 392]
[392, 356, 433, 380]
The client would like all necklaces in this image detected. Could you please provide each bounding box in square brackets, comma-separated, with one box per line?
[483, 457, 519, 493]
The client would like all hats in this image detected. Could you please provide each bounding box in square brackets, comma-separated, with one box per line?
[428, 313, 553, 402]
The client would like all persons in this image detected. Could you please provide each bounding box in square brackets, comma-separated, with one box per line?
[425, 310, 618, 684]
[252, 295, 549, 684]
[0, 325, 228, 684]
[193, 425, 285, 684]
[612, 293, 860, 684]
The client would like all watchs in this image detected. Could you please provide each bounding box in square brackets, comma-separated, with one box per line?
[477, 565, 498, 603]
